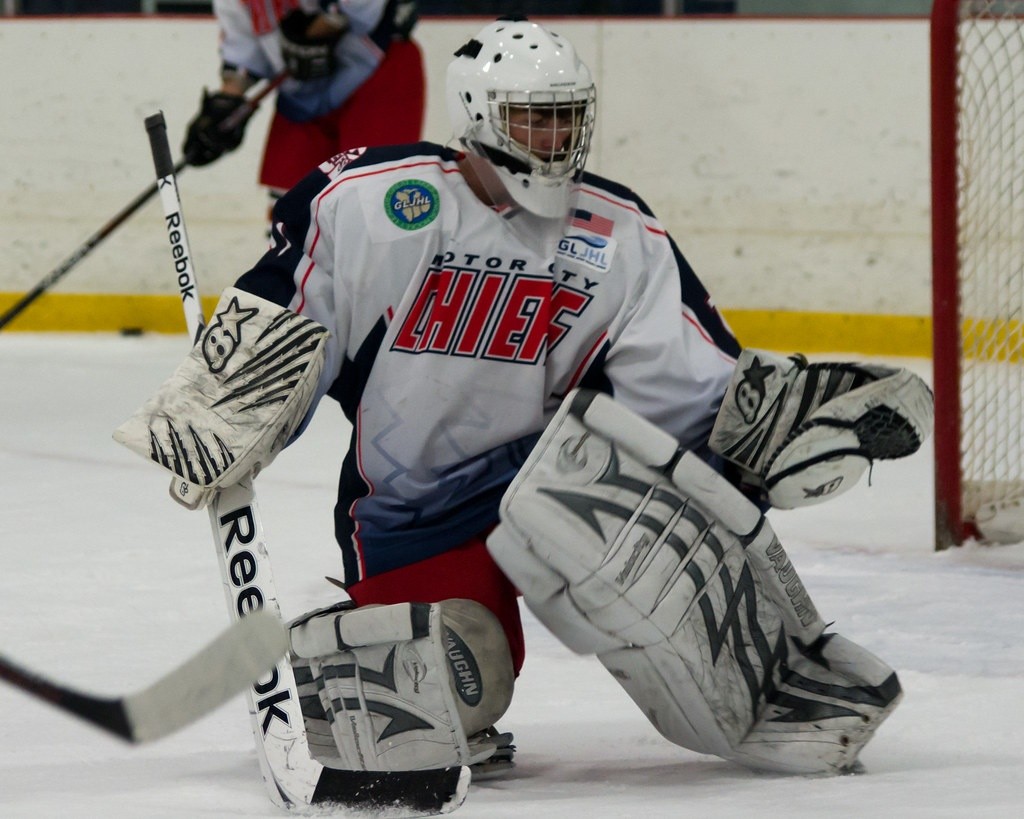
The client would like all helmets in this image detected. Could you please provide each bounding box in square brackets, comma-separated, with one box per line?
[449, 13, 595, 142]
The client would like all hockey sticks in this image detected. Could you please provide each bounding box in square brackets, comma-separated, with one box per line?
[0, 69, 471, 817]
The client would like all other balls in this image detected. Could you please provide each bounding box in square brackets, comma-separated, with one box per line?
[117, 326, 142, 335]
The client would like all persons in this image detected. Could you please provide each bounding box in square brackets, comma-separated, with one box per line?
[77, 0, 432, 199]
[108, 19, 939, 814]
